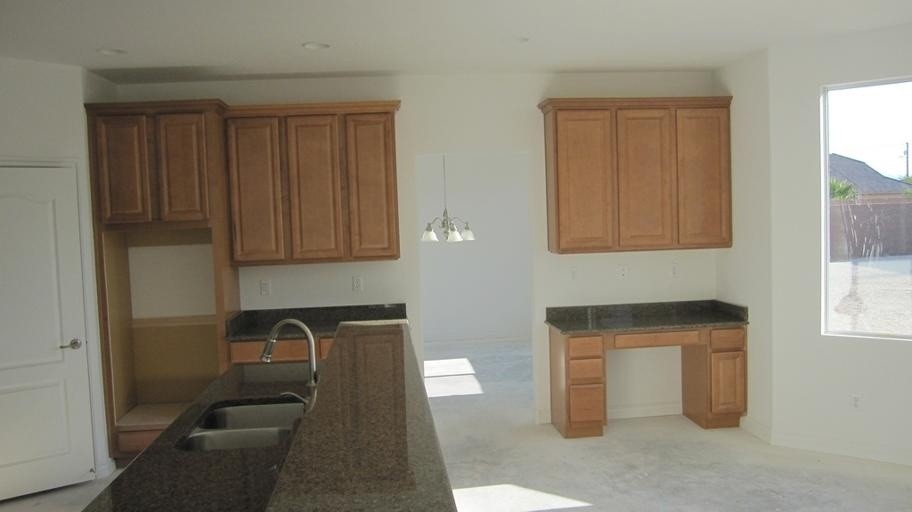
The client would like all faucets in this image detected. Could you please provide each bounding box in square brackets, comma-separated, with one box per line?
[259, 318, 319, 410]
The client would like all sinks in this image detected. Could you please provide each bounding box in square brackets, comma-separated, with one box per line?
[183, 428, 290, 453]
[195, 400, 307, 430]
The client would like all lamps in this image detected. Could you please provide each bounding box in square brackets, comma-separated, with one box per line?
[419, 155, 476, 245]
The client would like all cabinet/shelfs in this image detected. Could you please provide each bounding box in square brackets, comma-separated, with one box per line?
[537, 95, 734, 256]
[545, 322, 749, 440]
